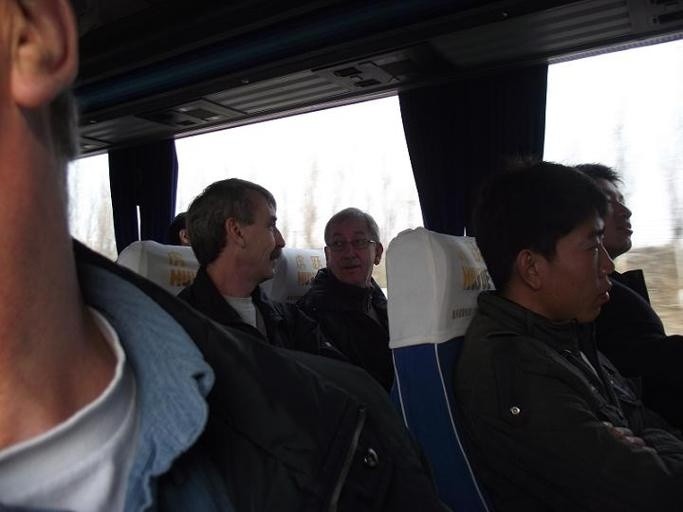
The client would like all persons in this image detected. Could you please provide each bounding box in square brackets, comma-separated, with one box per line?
[291, 204, 394, 400]
[165, 207, 192, 246]
[1, 0, 458, 512]
[561, 161, 682, 436]
[177, 174, 354, 365]
[454, 158, 682, 512]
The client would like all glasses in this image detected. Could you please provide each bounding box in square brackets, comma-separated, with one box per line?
[325, 238, 380, 250]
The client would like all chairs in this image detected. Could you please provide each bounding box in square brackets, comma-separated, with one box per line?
[387, 227, 495, 512]
[115, 239, 197, 296]
[261, 244, 327, 307]
